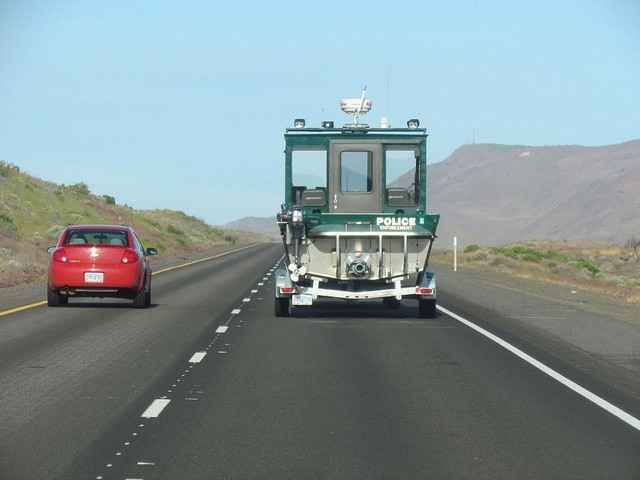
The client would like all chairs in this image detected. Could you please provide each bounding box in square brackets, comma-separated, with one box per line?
[110, 237, 124, 244]
[386, 187, 418, 205]
[69, 237, 85, 244]
[293, 187, 328, 207]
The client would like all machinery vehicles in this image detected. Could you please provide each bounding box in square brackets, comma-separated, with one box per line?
[274, 86, 441, 317]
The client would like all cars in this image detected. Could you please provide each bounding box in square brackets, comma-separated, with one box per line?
[43, 222, 158, 308]
